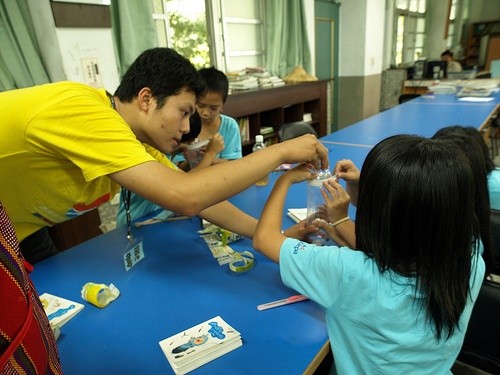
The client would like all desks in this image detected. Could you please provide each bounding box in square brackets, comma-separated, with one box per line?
[27, 79, 500, 375]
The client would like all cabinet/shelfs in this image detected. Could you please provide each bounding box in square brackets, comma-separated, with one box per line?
[220, 78, 336, 157]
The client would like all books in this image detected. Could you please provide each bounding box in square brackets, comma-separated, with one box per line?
[159, 316, 243, 375]
[38, 293, 85, 327]
[286, 208, 316, 223]
[228, 67, 285, 146]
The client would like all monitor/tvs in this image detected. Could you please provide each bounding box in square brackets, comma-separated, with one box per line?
[426, 61, 447, 79]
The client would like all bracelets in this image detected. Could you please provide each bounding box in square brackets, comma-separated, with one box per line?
[333, 216, 350, 227]
[281, 230, 284, 234]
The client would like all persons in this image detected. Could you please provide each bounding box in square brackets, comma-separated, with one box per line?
[440, 51, 461, 73]
[0, 46, 330, 268]
[251, 123, 500, 375]
[116, 67, 239, 230]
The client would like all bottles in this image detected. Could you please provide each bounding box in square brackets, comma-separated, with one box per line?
[251, 135, 270, 187]
[306, 165, 333, 246]
